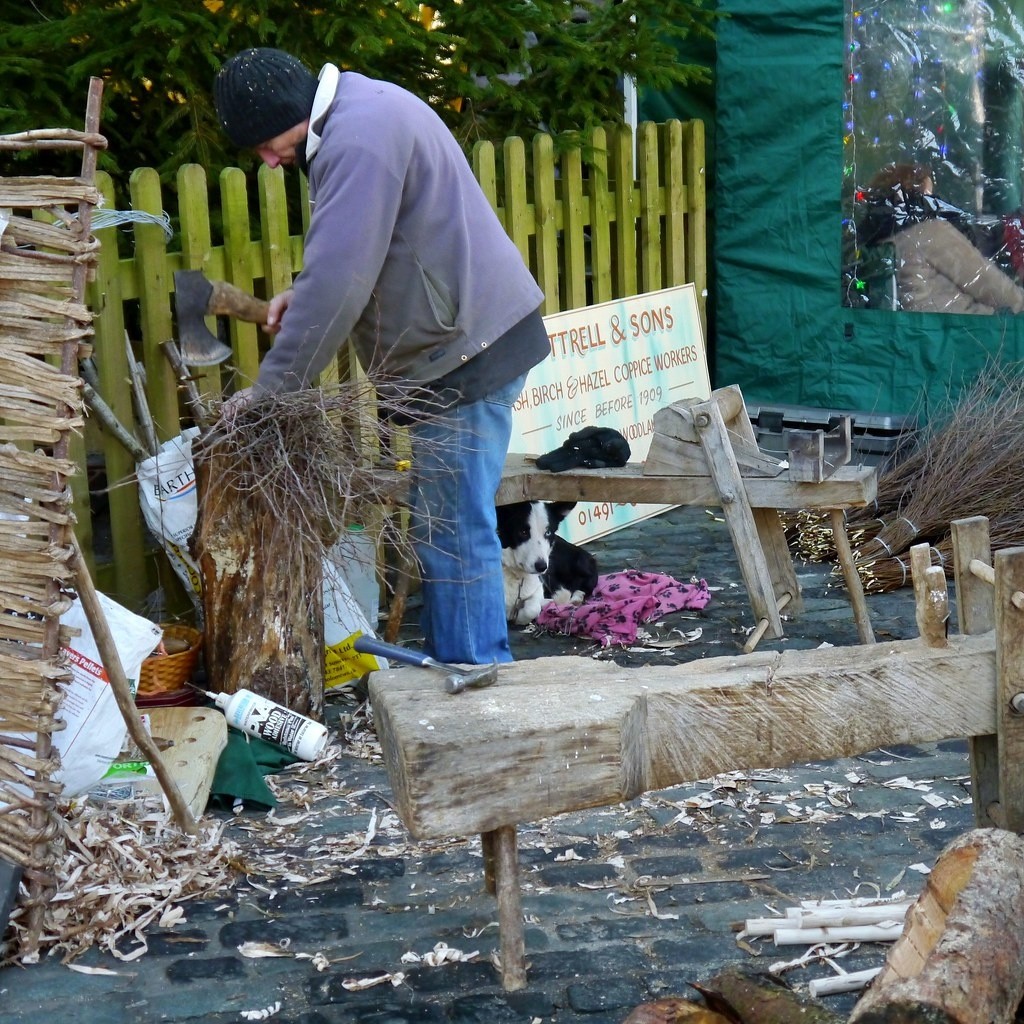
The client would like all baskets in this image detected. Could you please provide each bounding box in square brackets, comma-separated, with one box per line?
[136, 623, 202, 697]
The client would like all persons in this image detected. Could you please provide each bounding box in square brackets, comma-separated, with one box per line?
[852, 160, 1024, 317]
[213, 47, 554, 667]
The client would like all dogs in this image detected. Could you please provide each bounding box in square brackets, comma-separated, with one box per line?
[494, 502, 598, 628]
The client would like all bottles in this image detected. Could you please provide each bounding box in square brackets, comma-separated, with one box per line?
[205, 686, 330, 762]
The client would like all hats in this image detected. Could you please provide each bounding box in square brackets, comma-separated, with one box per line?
[216, 45, 321, 151]
